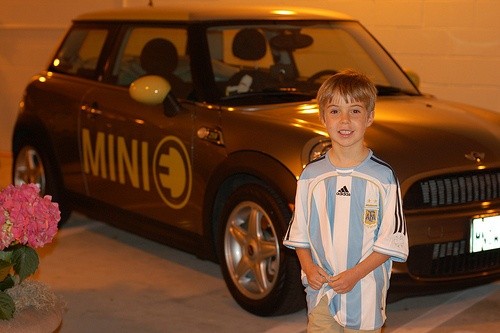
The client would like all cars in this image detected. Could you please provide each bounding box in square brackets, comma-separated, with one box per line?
[9, 6, 499, 321]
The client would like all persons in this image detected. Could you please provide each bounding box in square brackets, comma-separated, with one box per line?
[282, 68, 409, 332]
